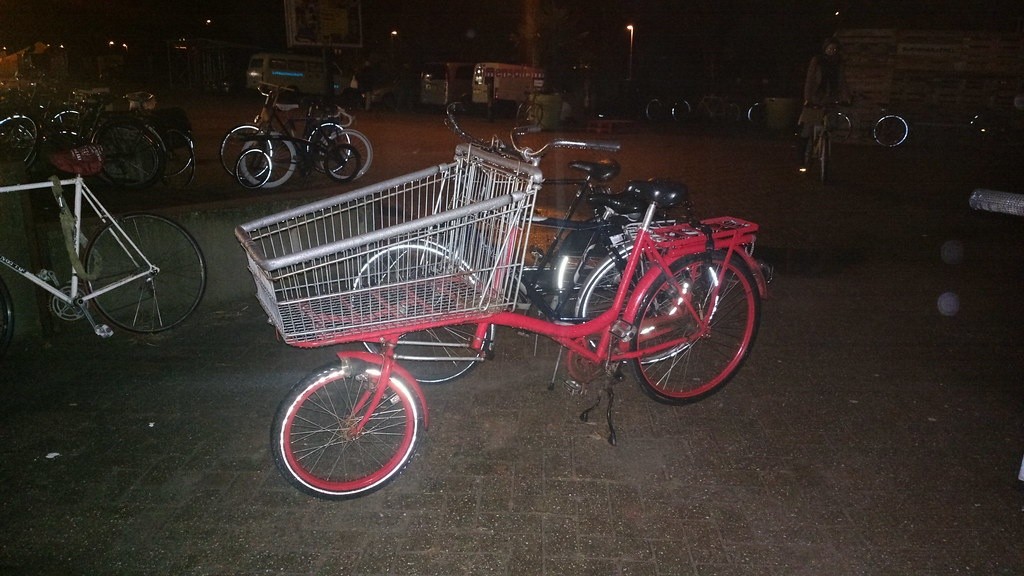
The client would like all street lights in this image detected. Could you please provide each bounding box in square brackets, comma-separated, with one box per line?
[626, 24, 634, 80]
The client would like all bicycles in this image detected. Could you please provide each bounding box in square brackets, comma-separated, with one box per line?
[801, 100, 840, 188]
[831, 88, 909, 147]
[224, 119, 775, 498]
[645, 96, 692, 122]
[1, 51, 376, 191]
[350, 121, 722, 389]
[358, 99, 694, 342]
[515, 87, 544, 126]
[0, 140, 208, 359]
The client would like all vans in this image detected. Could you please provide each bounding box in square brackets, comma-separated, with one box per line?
[420, 62, 474, 116]
[471, 62, 550, 116]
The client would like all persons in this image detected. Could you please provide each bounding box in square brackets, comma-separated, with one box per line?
[797, 36, 852, 171]
[359, 57, 377, 111]
[393, 60, 417, 113]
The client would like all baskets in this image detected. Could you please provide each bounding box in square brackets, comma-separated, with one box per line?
[234, 143, 543, 344]
